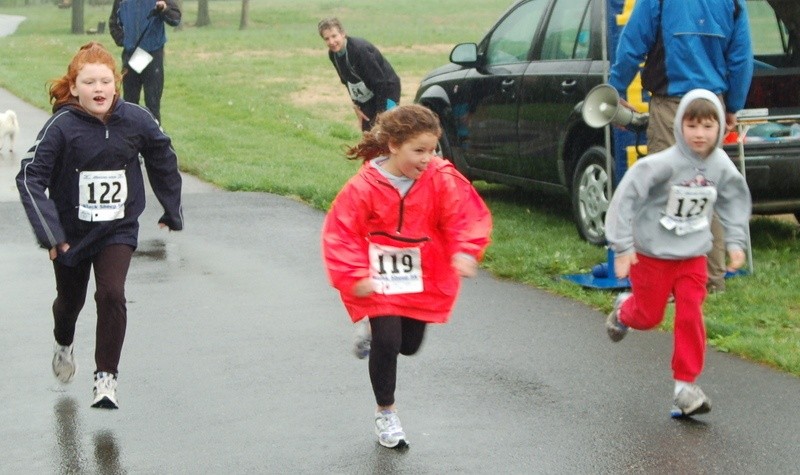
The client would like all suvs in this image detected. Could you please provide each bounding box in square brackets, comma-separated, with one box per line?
[414, 0, 800, 246]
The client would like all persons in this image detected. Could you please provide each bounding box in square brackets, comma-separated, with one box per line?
[604, 89, 753, 419]
[16, 41, 185, 408]
[318, 18, 400, 136]
[110, 0, 183, 166]
[322, 103, 493, 450]
[591, 1, 755, 281]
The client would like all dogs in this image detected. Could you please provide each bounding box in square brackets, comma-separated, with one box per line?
[0, 110, 17, 152]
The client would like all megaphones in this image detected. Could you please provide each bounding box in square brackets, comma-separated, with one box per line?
[581, 83, 650, 134]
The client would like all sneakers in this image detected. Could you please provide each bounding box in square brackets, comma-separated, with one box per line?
[374, 407, 409, 448]
[670, 382, 712, 417]
[353, 316, 373, 359]
[89, 372, 119, 410]
[605, 293, 629, 341]
[53, 340, 76, 382]
